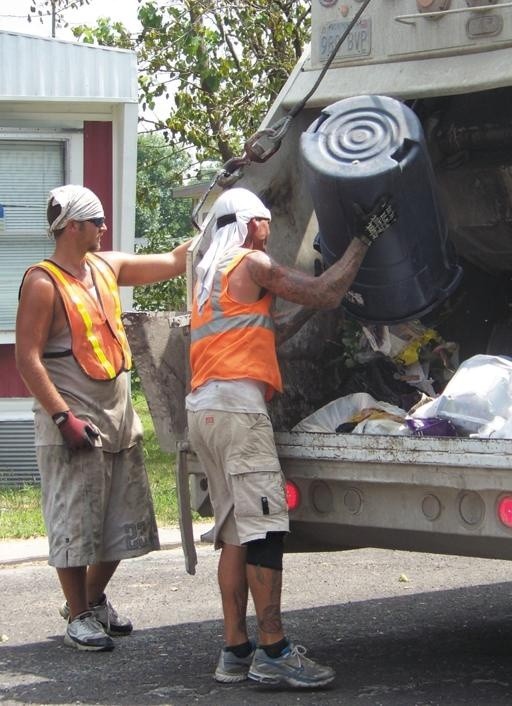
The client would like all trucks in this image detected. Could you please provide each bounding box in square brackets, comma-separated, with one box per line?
[118, 1, 512, 575]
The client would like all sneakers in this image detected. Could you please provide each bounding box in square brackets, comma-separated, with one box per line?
[59, 592, 134, 637]
[63, 613, 116, 652]
[214, 648, 253, 684]
[248, 641, 336, 689]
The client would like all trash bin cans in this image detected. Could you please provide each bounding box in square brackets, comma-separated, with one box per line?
[300, 94, 463, 323]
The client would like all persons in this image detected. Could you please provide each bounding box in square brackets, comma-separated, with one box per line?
[180, 187, 399, 690]
[13, 184, 194, 654]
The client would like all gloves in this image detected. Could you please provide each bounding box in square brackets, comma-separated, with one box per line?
[51, 408, 101, 456]
[348, 192, 399, 242]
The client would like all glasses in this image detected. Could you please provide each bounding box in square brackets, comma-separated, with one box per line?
[83, 217, 105, 227]
